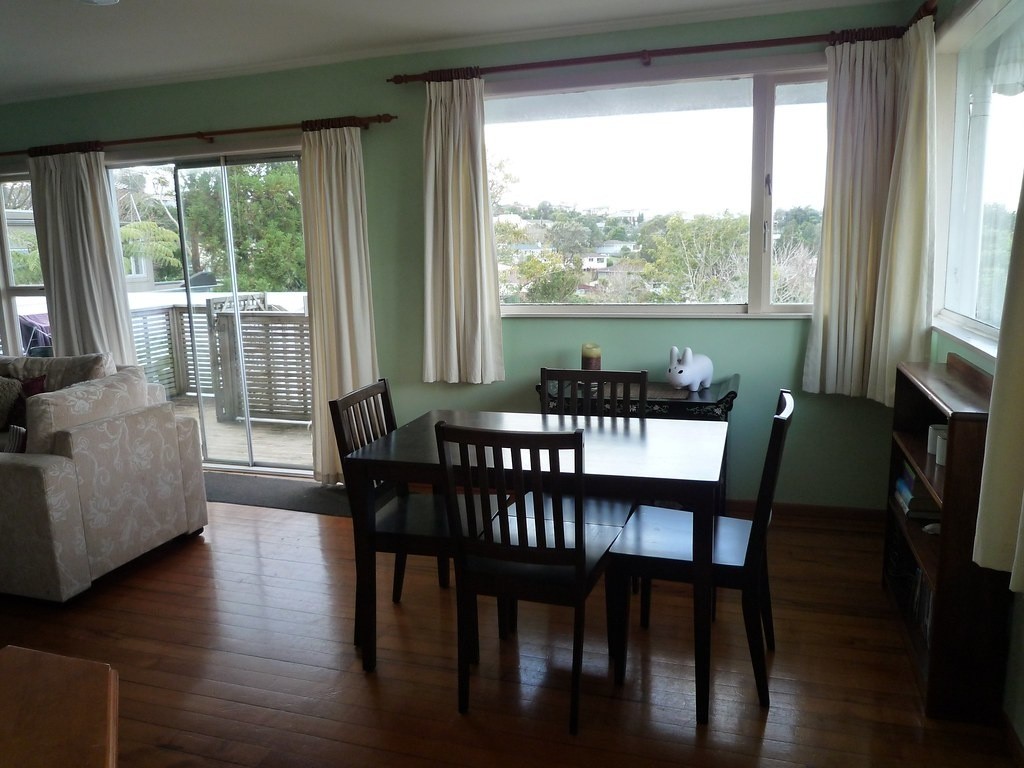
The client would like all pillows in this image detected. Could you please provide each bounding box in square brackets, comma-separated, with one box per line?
[4, 374, 46, 431]
[0, 376, 22, 432]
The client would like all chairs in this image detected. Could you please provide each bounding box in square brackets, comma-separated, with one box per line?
[327, 377, 512, 647]
[605, 388, 796, 708]
[506, 367, 648, 597]
[435, 420, 625, 736]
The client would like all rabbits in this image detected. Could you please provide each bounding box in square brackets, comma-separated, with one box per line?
[665, 345, 714, 391]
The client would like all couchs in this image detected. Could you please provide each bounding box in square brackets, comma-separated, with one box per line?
[0, 355, 209, 614]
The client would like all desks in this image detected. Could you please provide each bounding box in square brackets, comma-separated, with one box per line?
[343, 408, 730, 725]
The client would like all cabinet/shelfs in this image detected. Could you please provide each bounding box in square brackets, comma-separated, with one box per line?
[882, 351, 1017, 725]
[535, 373, 742, 516]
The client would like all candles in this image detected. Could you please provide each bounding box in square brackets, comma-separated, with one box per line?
[582, 343, 601, 370]
